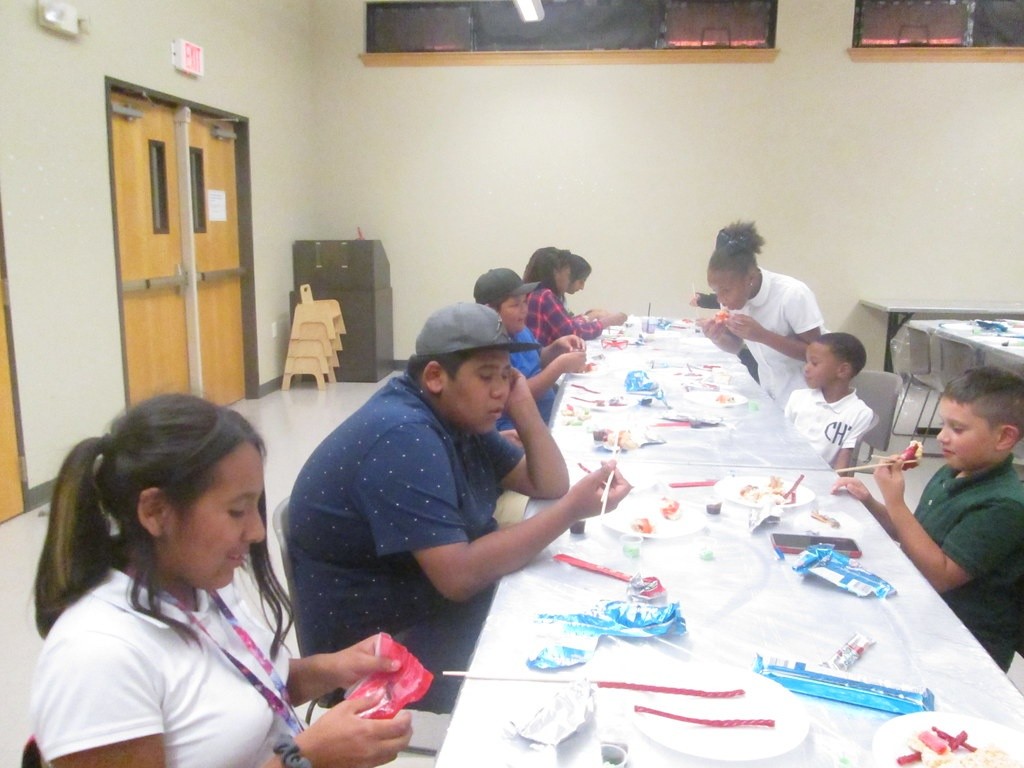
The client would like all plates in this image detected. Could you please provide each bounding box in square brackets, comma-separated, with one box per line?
[714, 474, 815, 508]
[872, 710, 1024, 768]
[626, 662, 810, 761]
[688, 390, 748, 408]
[603, 498, 707, 537]
[588, 392, 640, 410]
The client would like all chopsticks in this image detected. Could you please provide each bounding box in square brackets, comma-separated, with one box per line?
[599, 434, 621, 517]
[836, 455, 918, 472]
[442, 671, 572, 681]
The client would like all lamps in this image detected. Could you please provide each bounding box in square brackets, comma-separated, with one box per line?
[35, 0, 92, 38]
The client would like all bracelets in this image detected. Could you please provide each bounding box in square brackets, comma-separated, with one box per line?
[272, 734, 312, 768]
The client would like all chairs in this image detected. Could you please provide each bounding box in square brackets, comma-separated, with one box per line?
[273, 496, 449, 755]
[892, 326, 975, 458]
[848, 370, 903, 477]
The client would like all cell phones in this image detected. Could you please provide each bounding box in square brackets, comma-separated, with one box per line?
[771, 533, 862, 558]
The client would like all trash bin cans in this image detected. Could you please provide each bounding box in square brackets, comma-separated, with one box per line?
[289, 238, 395, 384]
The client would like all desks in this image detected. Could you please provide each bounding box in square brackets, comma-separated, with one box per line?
[435, 329, 1024, 768]
[859, 297, 1024, 373]
[928, 322, 1024, 363]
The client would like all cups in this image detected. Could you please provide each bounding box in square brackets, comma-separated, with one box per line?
[641, 316, 656, 337]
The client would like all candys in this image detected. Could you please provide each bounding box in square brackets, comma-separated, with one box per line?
[818, 630, 875, 670]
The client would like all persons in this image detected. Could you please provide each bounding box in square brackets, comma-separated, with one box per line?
[288, 301, 631, 713]
[473, 246, 628, 430]
[830, 368, 1024, 675]
[690, 222, 825, 410]
[785, 333, 873, 477]
[32, 394, 413, 768]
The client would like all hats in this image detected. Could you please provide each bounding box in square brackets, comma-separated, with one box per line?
[474, 268, 542, 304]
[415, 301, 541, 357]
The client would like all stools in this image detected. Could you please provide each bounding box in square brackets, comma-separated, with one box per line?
[281, 298, 347, 391]
